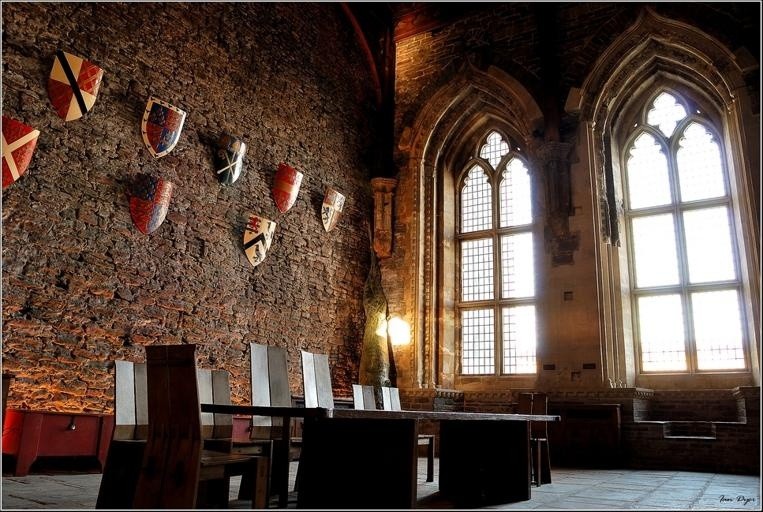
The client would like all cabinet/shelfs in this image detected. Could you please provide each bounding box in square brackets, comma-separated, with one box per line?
[1, 404, 304, 477]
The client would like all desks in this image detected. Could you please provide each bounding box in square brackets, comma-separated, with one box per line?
[200, 404, 560, 508]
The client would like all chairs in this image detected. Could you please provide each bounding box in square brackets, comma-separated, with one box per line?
[95, 359, 147, 510]
[515, 392, 552, 487]
[197, 368, 275, 507]
[129, 347, 267, 508]
[294, 348, 335, 489]
[382, 386, 435, 483]
[238, 341, 302, 503]
[351, 384, 376, 409]
